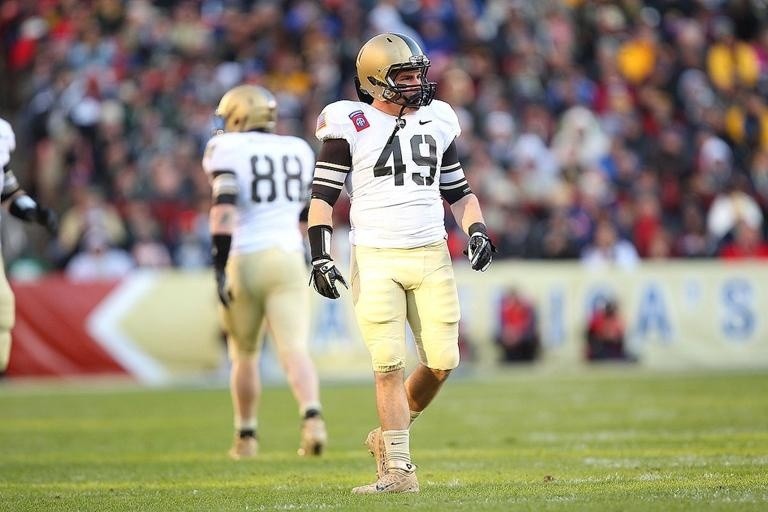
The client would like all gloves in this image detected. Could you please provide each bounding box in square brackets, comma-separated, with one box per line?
[468, 233, 492, 272]
[216, 268, 233, 307]
[28, 204, 57, 235]
[313, 260, 348, 298]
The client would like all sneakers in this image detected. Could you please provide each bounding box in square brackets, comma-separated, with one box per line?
[351, 460, 418, 496]
[304, 409, 328, 457]
[368, 428, 386, 477]
[228, 431, 256, 461]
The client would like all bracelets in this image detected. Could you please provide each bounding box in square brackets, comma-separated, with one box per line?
[212, 235, 231, 269]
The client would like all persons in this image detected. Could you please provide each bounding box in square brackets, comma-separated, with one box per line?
[331, 34, 498, 496]
[200, 84, 326, 461]
[0, 1, 768, 260]
[0, 117, 57, 380]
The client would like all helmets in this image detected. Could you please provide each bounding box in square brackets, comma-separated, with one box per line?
[215, 84, 278, 132]
[356, 34, 437, 109]
